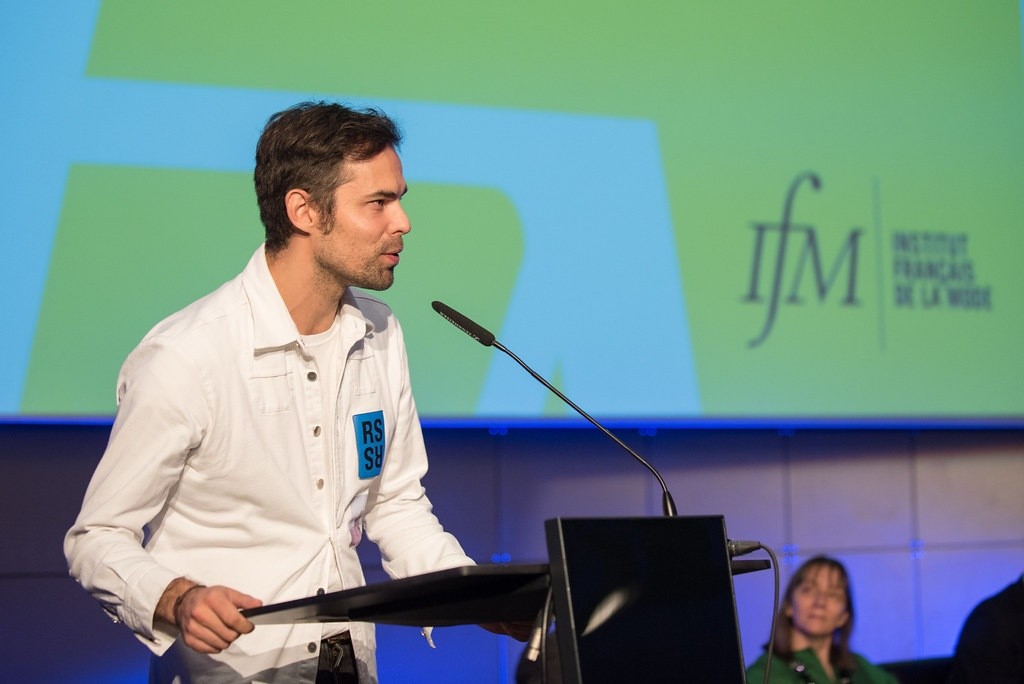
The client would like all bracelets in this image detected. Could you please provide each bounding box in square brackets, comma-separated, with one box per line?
[173, 585, 207, 624]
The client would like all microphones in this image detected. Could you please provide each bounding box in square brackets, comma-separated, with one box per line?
[432, 300, 677, 517]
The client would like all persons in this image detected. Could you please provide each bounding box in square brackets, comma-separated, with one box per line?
[744, 555, 890, 684]
[62, 99, 550, 684]
